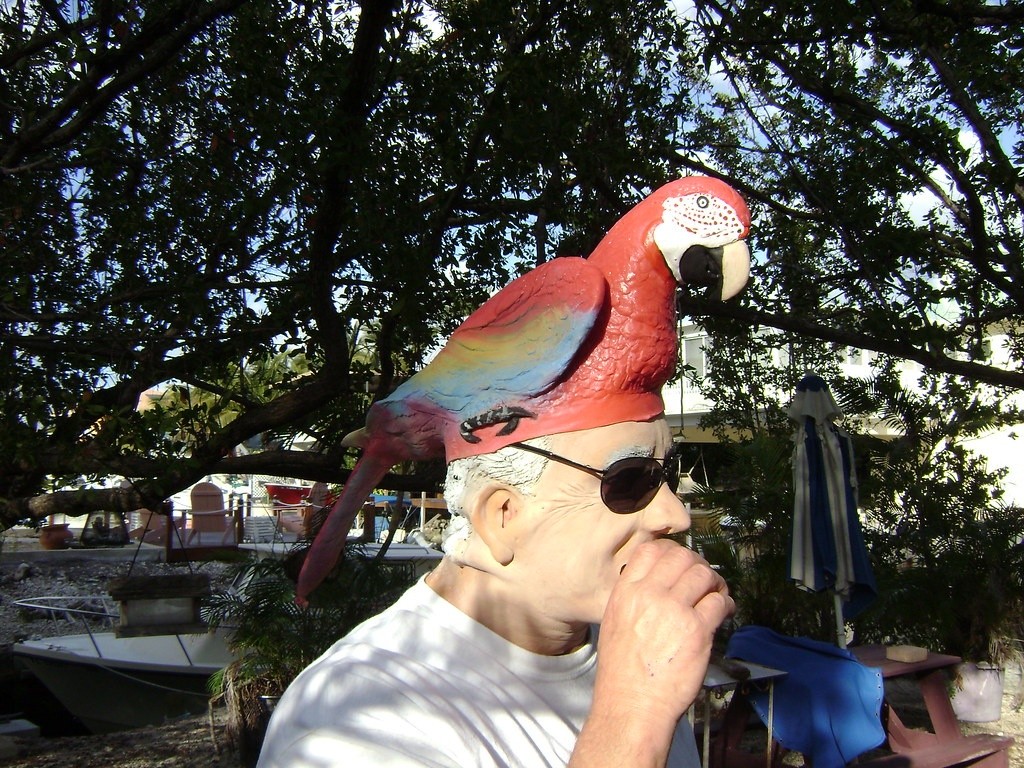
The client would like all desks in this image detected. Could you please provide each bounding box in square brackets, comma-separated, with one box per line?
[708, 644, 961, 768]
[688, 660, 788, 768]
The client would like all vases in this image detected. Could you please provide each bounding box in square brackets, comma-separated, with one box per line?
[39, 523, 74, 550]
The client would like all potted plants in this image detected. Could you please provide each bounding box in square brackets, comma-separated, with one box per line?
[947, 547, 1016, 723]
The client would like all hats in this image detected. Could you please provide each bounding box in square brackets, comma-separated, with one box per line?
[293, 174, 752, 607]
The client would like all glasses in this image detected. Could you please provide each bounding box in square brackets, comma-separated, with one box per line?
[512, 440, 682, 515]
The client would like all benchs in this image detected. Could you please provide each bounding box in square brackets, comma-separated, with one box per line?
[840, 733, 1016, 768]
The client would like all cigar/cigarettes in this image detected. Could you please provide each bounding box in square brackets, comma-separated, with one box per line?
[709, 564, 726, 570]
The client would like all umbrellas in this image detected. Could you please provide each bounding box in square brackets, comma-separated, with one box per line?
[786, 369, 876, 650]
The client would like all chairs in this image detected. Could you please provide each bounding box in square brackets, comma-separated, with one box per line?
[187, 483, 239, 544]
[260, 501, 313, 557]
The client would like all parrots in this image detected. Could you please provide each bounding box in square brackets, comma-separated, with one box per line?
[297, 176, 750, 597]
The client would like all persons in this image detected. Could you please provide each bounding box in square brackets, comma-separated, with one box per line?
[257, 412, 737, 768]
